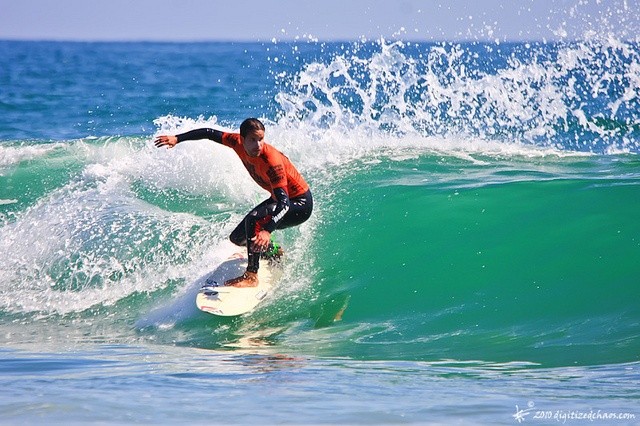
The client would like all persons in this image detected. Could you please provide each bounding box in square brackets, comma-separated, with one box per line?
[153, 116, 315, 290]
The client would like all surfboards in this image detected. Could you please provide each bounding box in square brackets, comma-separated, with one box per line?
[195, 246, 281, 316]
